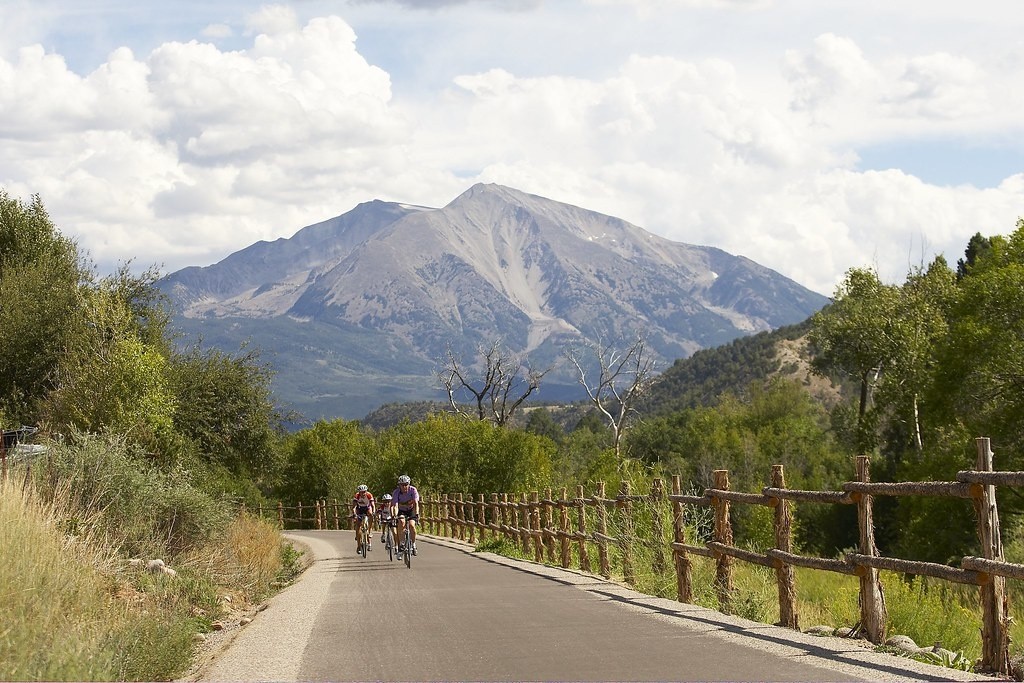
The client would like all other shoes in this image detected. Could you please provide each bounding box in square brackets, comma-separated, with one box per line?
[381, 536, 385, 543]
[357, 547, 361, 554]
[368, 543, 372, 551]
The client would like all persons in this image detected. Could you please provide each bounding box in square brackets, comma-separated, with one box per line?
[376, 493, 398, 553]
[393, 475, 420, 560]
[353, 485, 376, 554]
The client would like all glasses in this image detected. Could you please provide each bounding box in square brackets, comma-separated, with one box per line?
[400, 483, 409, 487]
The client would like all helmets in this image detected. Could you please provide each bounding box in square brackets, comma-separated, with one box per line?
[397, 475, 411, 484]
[358, 485, 368, 492]
[382, 494, 393, 500]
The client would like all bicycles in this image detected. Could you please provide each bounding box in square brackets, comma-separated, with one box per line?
[379, 519, 396, 562]
[350, 514, 377, 558]
[390, 514, 419, 569]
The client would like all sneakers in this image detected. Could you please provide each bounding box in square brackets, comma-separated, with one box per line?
[412, 546, 418, 556]
[397, 552, 402, 560]
